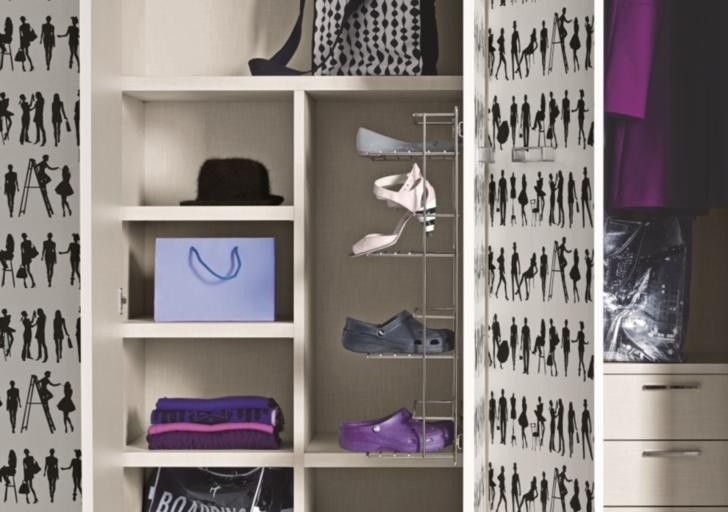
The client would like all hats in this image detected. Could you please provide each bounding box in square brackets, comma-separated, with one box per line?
[178, 156, 285, 204]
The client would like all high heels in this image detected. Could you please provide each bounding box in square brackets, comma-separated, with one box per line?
[346, 161, 439, 258]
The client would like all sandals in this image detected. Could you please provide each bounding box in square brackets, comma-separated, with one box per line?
[340, 307, 456, 355]
[336, 407, 455, 455]
[354, 124, 458, 155]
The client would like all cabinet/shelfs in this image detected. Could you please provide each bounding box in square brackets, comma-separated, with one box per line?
[0, 0, 604, 512]
[603, 359, 728, 512]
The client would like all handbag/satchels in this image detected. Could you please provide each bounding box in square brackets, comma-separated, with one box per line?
[248, 1, 442, 77]
[138, 466, 282, 512]
[153, 235, 279, 323]
